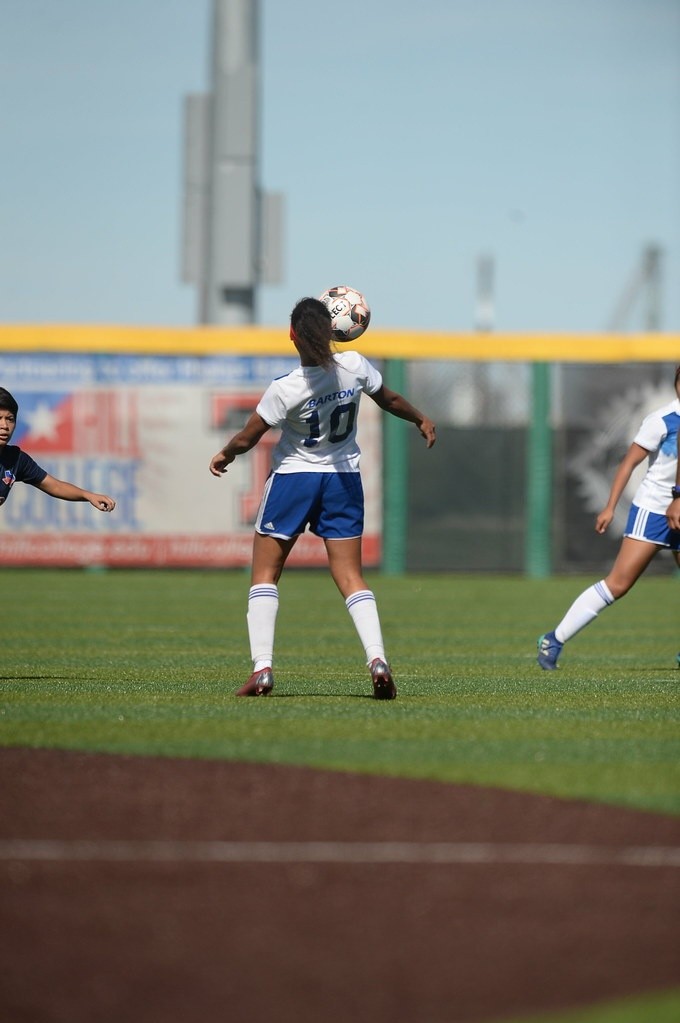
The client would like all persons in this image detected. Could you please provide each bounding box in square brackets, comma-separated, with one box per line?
[209, 298, 436, 700]
[0, 387, 116, 512]
[537, 366, 680, 671]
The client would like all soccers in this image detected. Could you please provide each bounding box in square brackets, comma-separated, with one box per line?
[316, 285, 372, 343]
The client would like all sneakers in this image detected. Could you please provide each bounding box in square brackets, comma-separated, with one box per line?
[235, 666, 274, 696]
[537, 631, 563, 670]
[371, 658, 397, 699]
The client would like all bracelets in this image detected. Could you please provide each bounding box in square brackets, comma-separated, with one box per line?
[672, 486, 680, 498]
